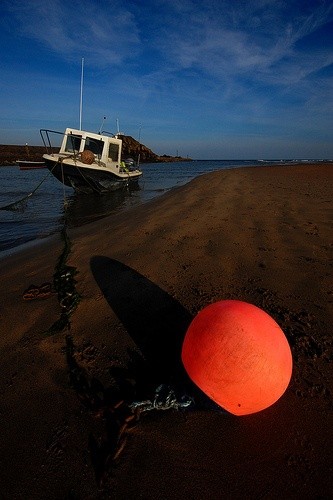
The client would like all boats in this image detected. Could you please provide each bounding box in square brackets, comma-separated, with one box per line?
[15, 141, 47, 170]
[39, 57, 143, 194]
